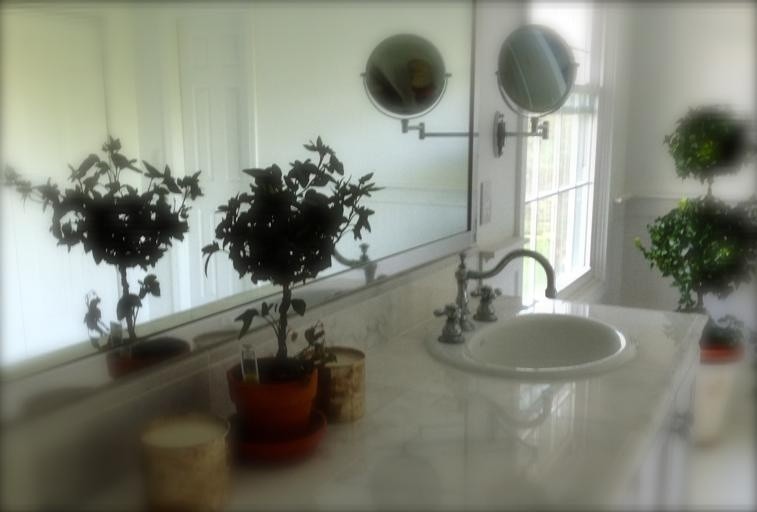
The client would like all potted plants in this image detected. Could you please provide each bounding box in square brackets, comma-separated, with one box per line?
[194, 133, 387, 446]
[629, 101, 757, 448]
[0, 131, 206, 381]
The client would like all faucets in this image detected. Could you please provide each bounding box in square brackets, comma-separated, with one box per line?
[331, 243, 378, 281]
[455, 249, 556, 329]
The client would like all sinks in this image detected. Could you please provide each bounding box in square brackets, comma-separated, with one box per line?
[425, 307, 627, 375]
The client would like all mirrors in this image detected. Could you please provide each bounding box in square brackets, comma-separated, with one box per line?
[490, 23, 579, 159]
[0, 0, 479, 384]
[358, 32, 471, 142]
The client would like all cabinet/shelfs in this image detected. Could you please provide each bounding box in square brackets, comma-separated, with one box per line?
[616, 342, 700, 510]
